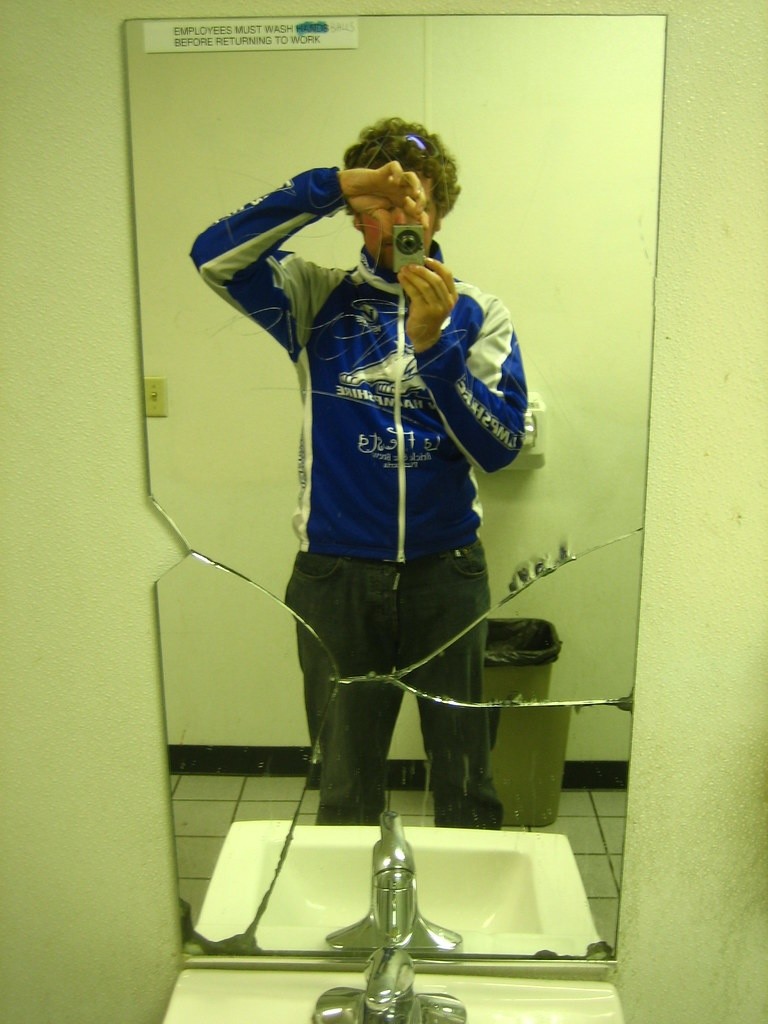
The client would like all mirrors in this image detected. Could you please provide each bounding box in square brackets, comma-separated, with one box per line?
[121, 13, 668, 967]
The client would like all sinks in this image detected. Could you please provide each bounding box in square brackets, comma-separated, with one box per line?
[194, 821, 598, 944]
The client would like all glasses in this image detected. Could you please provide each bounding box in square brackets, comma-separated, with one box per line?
[363, 134, 440, 163]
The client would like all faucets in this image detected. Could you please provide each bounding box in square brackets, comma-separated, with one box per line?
[326, 811, 463, 953]
[313, 945, 468, 1024]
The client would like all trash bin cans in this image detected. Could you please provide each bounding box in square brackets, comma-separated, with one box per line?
[482, 617, 568, 828]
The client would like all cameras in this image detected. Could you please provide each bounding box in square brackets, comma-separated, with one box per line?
[392, 224, 425, 272]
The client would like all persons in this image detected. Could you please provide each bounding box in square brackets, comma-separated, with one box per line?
[189, 118, 527, 831]
